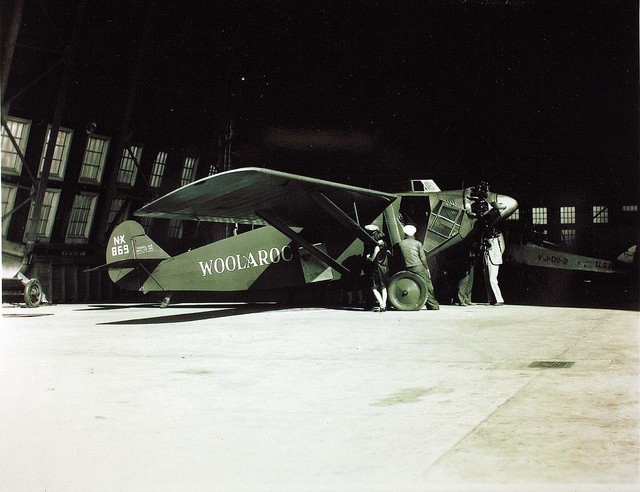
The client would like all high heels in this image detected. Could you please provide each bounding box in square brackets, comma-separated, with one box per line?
[374, 307, 385, 312]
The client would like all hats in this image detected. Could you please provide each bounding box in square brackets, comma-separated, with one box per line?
[402, 225, 417, 237]
[364, 225, 378, 232]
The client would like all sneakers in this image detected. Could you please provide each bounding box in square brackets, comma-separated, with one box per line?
[467, 302, 475, 306]
[459, 302, 467, 306]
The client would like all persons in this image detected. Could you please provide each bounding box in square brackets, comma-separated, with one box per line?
[480, 226, 505, 306]
[451, 242, 477, 306]
[366, 231, 390, 312]
[347, 288, 364, 306]
[365, 225, 378, 236]
[399, 225, 439, 309]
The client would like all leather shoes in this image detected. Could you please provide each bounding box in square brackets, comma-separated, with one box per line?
[495, 303, 503, 306]
